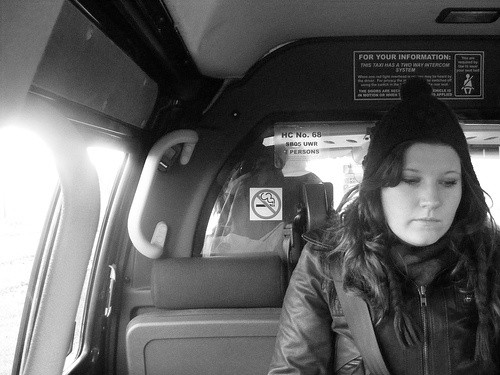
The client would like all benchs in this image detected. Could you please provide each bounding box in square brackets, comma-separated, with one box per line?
[124, 252, 288, 375]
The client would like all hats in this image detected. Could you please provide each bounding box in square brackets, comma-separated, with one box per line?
[364, 77, 470, 178]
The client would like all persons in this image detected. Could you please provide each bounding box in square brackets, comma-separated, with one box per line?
[265, 74, 500, 374]
